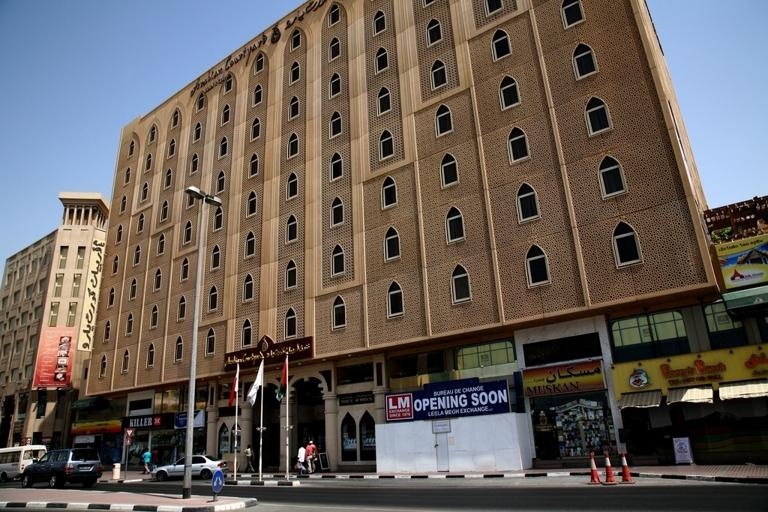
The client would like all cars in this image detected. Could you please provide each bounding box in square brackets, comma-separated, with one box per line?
[149, 453, 229, 483]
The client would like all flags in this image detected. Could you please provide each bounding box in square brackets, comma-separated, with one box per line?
[227, 363, 239, 406]
[246, 359, 262, 407]
[275, 356, 287, 402]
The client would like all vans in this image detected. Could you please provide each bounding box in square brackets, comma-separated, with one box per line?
[0, 444, 50, 482]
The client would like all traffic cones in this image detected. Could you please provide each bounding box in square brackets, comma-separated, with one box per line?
[586, 451, 602, 485]
[618, 451, 638, 485]
[601, 448, 618, 487]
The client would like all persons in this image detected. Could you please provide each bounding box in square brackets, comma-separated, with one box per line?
[306, 440, 318, 473]
[297, 442, 306, 474]
[244, 444, 256, 472]
[142, 448, 152, 474]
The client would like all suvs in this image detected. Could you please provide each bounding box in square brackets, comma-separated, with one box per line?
[20, 447, 106, 490]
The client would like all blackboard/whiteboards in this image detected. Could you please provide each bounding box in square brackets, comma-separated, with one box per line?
[319, 453, 329, 470]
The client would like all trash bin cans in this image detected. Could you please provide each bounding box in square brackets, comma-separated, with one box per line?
[112, 463, 121, 479]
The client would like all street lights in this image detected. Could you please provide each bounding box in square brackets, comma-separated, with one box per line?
[180, 183, 224, 499]
